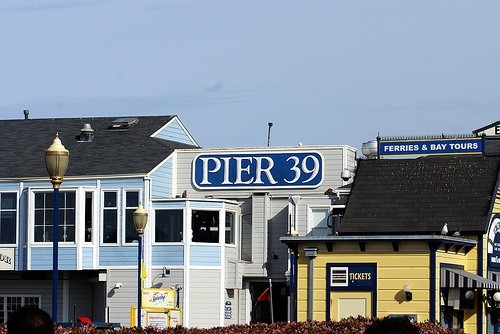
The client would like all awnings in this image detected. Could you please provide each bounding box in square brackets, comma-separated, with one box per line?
[440, 267, 500, 289]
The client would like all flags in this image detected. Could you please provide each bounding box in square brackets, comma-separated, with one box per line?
[257, 287, 270, 302]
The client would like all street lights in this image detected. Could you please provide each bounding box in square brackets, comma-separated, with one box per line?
[44, 131, 70, 324]
[132, 202, 148, 327]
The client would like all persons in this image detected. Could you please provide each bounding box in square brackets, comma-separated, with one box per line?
[6, 305, 54, 334]
